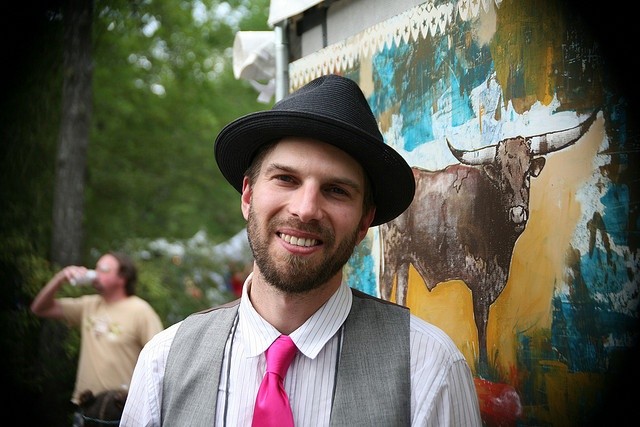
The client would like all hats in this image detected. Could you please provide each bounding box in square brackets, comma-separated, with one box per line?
[212, 74, 416, 228]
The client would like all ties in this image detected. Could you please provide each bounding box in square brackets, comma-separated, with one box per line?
[251, 335, 299, 427]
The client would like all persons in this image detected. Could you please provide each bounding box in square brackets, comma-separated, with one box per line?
[119, 74, 483, 426]
[30, 249, 164, 426]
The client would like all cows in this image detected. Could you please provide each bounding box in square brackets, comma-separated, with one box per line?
[379, 107, 601, 379]
